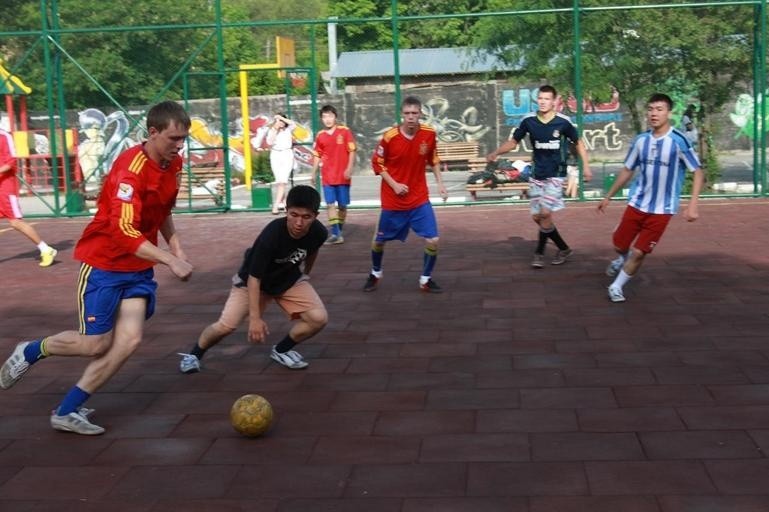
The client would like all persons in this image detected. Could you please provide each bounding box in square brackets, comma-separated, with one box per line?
[265, 113, 297, 215]
[681, 104, 697, 143]
[596, 93, 705, 303]
[1, 100, 196, 436]
[485, 84, 593, 268]
[1, 112, 59, 268]
[309, 104, 357, 245]
[363, 96, 450, 293]
[177, 185, 330, 374]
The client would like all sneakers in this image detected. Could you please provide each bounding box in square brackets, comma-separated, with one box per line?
[608, 283, 625, 303]
[50, 407, 106, 435]
[176, 352, 201, 374]
[38, 247, 58, 267]
[270, 344, 309, 370]
[0, 340, 31, 392]
[530, 254, 545, 268]
[551, 247, 574, 265]
[322, 234, 345, 245]
[418, 274, 444, 293]
[362, 268, 383, 293]
[605, 258, 624, 277]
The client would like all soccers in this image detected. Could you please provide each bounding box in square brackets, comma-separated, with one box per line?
[230, 394, 276, 439]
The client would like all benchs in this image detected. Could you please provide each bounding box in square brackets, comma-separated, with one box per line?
[425, 140, 480, 172]
[177, 167, 227, 206]
[466, 156, 568, 201]
